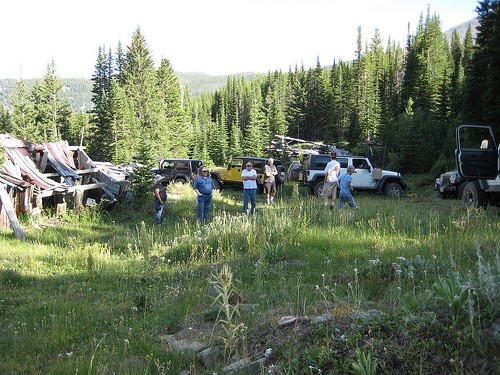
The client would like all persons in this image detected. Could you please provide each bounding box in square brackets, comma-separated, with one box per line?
[194, 167, 215, 219]
[153, 174, 167, 225]
[240, 162, 258, 212]
[321, 151, 341, 208]
[336, 165, 358, 209]
[262, 157, 278, 205]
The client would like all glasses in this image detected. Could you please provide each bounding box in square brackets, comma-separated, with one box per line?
[247, 165, 251, 167]
[202, 171, 208, 172]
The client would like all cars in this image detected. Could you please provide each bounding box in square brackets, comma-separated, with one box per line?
[433, 170, 464, 200]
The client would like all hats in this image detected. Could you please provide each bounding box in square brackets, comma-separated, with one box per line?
[154, 175, 164, 182]
[329, 151, 336, 157]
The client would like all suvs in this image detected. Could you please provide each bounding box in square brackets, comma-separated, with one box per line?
[210, 156, 286, 196]
[454, 123, 500, 213]
[150, 159, 223, 191]
[291, 152, 407, 201]
